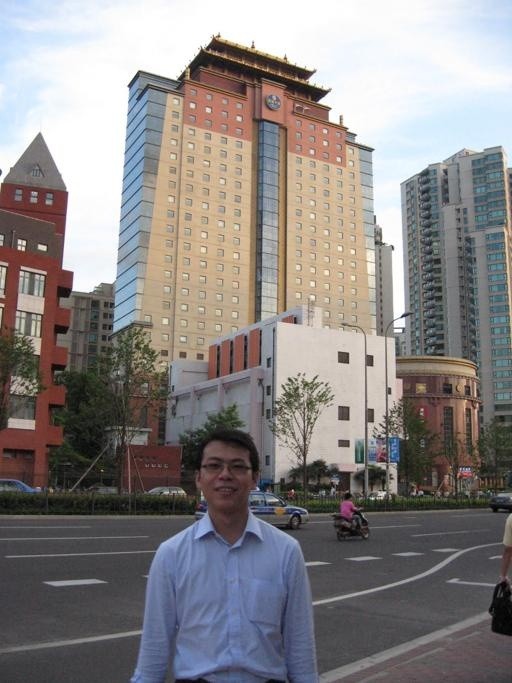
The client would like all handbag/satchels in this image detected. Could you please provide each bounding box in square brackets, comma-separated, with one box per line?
[488, 578, 512, 636]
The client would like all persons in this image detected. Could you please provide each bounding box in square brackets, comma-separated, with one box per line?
[409, 486, 418, 497]
[129, 429, 320, 683]
[340, 493, 364, 529]
[500, 514, 512, 602]
[288, 487, 296, 501]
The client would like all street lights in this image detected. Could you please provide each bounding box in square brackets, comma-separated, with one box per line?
[341, 323, 368, 498]
[384, 312, 414, 497]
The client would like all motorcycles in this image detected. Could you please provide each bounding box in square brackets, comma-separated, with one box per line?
[330, 505, 369, 541]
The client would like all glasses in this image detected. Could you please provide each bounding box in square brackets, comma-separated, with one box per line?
[199, 461, 256, 475]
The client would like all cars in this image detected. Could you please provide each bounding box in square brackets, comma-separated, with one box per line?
[147, 487, 186, 496]
[489, 492, 512, 512]
[0, 478, 37, 493]
[360, 491, 391, 503]
[195, 491, 309, 529]
